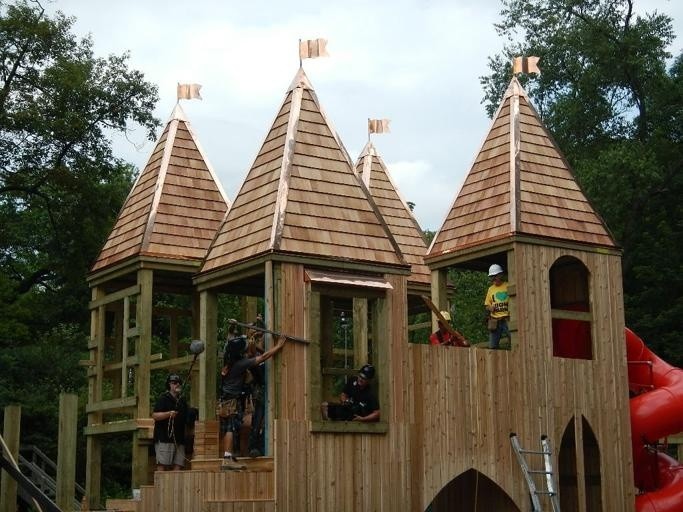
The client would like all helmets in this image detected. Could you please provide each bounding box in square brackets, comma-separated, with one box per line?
[436, 311, 451, 322]
[488, 263, 503, 277]
[358, 364, 376, 380]
[226, 337, 248, 354]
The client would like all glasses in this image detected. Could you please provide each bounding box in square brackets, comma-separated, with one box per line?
[172, 381, 182, 385]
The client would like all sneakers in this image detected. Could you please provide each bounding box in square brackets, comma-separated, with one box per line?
[221, 457, 246, 469]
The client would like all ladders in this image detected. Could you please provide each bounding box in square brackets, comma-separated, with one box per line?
[509, 432, 559, 512]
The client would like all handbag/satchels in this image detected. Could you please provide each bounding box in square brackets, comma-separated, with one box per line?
[487, 318, 498, 330]
[216, 398, 238, 417]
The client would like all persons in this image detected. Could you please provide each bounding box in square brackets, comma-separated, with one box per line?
[151, 372, 191, 471]
[320, 363, 378, 421]
[483, 264, 510, 349]
[216, 313, 288, 470]
[429, 311, 469, 347]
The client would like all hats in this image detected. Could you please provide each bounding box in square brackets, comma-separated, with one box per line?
[169, 374, 181, 381]
